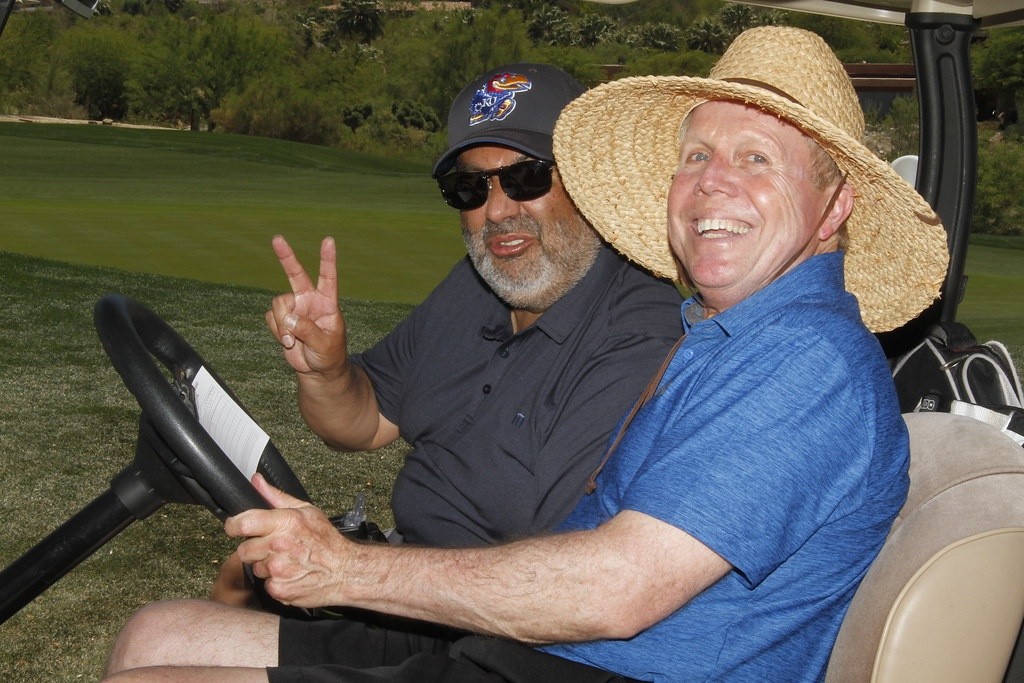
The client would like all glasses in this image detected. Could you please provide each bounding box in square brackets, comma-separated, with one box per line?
[437, 159, 553, 210]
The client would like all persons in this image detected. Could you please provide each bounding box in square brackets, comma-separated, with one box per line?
[210, 65, 687, 613]
[101, 25, 910, 683]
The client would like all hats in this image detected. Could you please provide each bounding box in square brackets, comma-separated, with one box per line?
[552, 26, 950, 338]
[432, 61, 586, 177]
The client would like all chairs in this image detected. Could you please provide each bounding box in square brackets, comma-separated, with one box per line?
[824, 411, 1024, 683]
